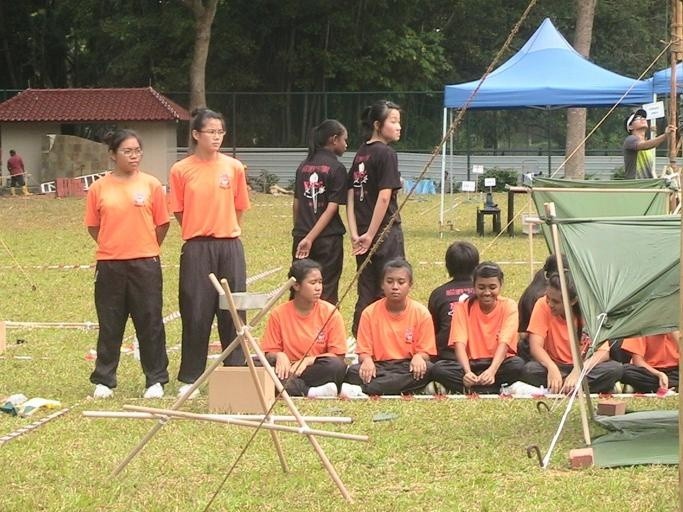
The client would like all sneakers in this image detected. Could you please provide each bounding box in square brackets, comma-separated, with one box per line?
[144, 383, 165, 399]
[518, 381, 544, 396]
[411, 382, 436, 394]
[340, 382, 362, 398]
[177, 383, 201, 400]
[607, 381, 623, 395]
[307, 381, 338, 398]
[94, 383, 114, 398]
[499, 380, 518, 397]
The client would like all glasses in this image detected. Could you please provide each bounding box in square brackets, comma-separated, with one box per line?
[119, 149, 144, 157]
[199, 128, 228, 137]
[634, 116, 645, 121]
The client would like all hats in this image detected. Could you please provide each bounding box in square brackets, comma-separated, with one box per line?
[626, 109, 646, 132]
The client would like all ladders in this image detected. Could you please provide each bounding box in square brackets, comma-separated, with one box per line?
[40, 171, 113, 194]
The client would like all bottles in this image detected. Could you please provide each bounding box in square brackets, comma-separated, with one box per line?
[486, 192, 493, 209]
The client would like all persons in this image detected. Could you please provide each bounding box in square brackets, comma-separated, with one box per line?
[288, 118, 348, 311]
[623, 109, 678, 179]
[346, 99, 405, 341]
[246, 259, 348, 398]
[170, 106, 252, 399]
[7, 150, 33, 196]
[428, 240, 680, 395]
[84, 128, 170, 398]
[339, 256, 437, 399]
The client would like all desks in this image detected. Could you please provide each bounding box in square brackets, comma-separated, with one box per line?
[405, 179, 434, 194]
[504, 185, 530, 236]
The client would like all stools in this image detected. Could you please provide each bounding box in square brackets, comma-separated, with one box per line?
[477, 207, 501, 235]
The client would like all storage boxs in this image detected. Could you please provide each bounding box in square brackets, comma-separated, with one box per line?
[207, 367, 275, 415]
